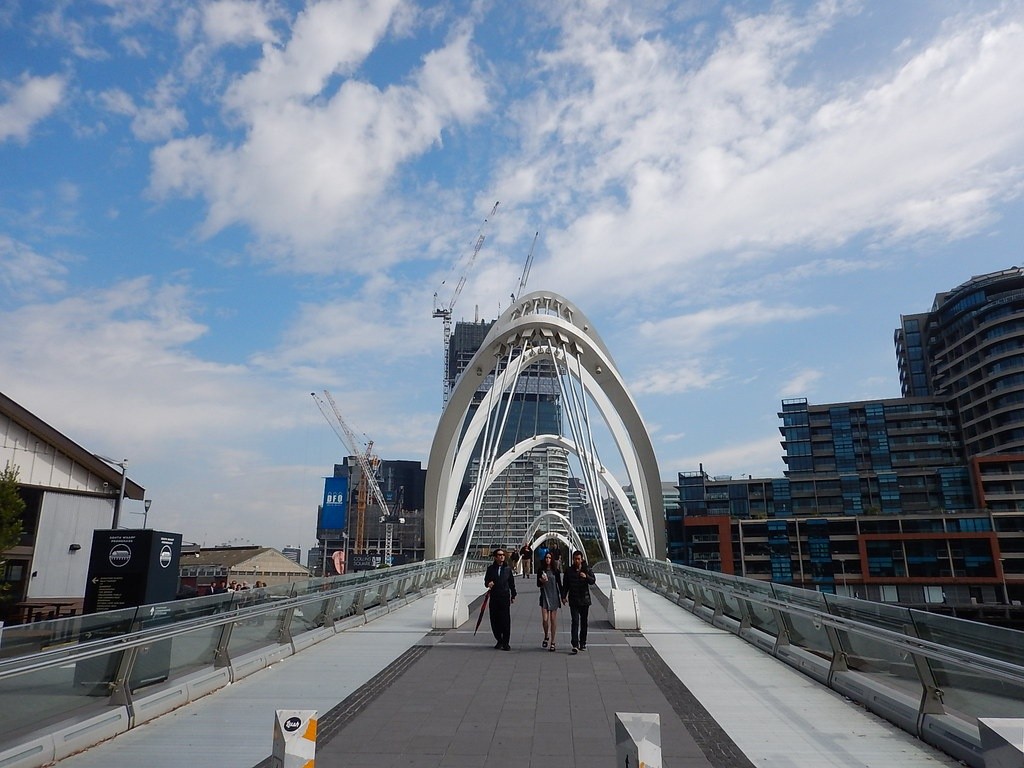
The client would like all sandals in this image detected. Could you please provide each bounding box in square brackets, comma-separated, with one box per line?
[550, 642, 556, 651]
[542, 637, 549, 648]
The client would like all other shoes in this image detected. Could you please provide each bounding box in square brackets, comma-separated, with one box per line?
[580, 644, 587, 650]
[503, 643, 511, 650]
[494, 642, 503, 649]
[527, 575, 529, 578]
[523, 575, 525, 578]
[572, 647, 580, 653]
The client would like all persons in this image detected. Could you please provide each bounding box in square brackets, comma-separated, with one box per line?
[485, 549, 517, 651]
[520, 542, 533, 578]
[206, 581, 267, 627]
[537, 550, 596, 653]
[537, 543, 549, 569]
[511, 549, 520, 577]
[551, 543, 562, 570]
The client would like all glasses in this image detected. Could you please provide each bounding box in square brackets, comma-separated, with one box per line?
[496, 554, 505, 556]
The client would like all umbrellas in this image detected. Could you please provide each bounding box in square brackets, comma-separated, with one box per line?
[474, 584, 491, 635]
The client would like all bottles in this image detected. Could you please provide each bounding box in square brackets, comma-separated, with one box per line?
[541, 571, 549, 582]
